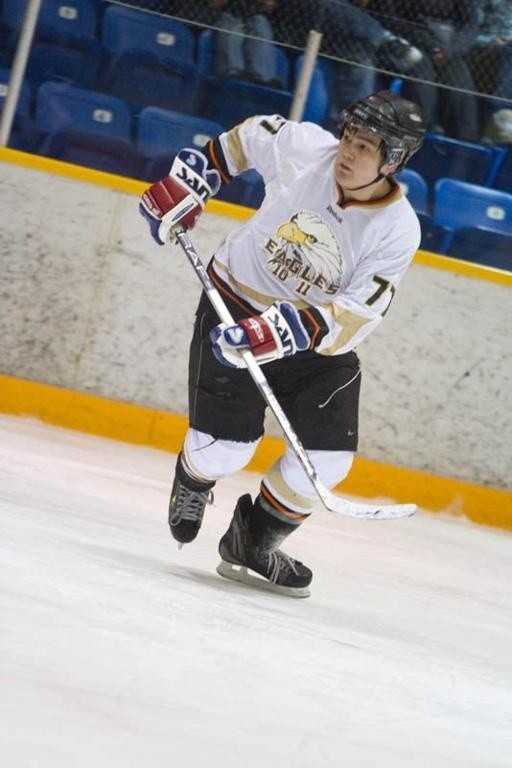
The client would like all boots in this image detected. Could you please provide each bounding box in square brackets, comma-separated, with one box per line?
[218, 494, 312, 588]
[168, 453, 216, 543]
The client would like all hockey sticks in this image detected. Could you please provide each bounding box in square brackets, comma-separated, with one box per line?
[172, 225, 418, 521]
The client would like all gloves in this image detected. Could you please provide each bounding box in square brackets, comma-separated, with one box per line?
[138, 149, 222, 245]
[209, 301, 310, 369]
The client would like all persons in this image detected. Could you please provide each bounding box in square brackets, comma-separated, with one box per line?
[188, 1, 511, 182]
[135, 88, 428, 591]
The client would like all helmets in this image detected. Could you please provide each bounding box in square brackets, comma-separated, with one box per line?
[339, 89, 428, 175]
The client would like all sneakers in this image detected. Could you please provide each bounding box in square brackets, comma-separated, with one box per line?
[376, 37, 422, 71]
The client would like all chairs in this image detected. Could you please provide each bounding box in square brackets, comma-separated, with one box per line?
[0, 1, 511, 272]
